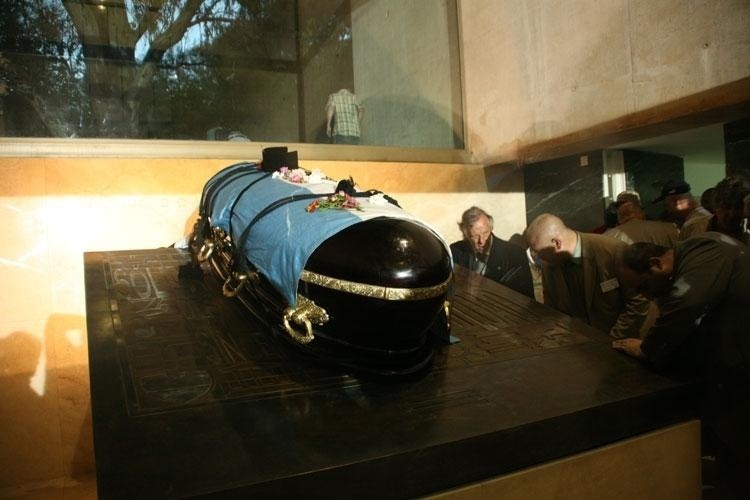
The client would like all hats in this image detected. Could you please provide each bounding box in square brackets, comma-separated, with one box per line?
[651, 181, 690, 204]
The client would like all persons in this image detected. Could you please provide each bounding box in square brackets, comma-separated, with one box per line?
[524, 172, 750, 500]
[448, 206, 537, 302]
[323, 79, 367, 146]
